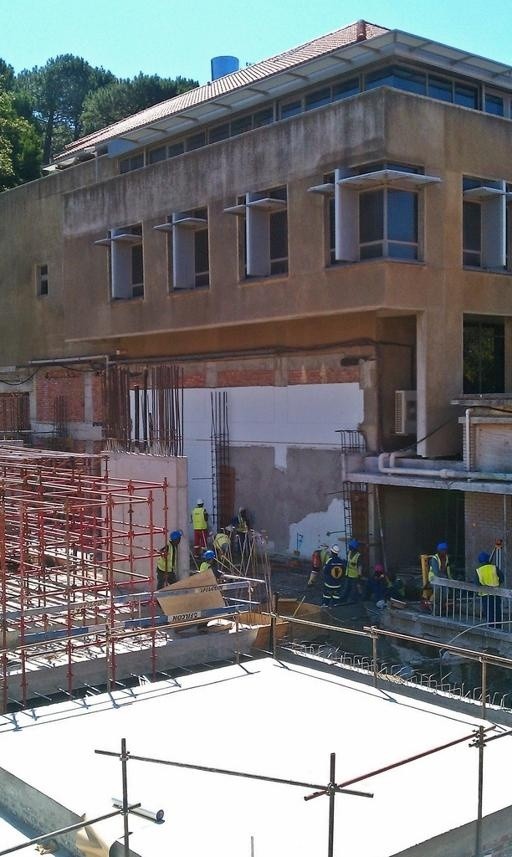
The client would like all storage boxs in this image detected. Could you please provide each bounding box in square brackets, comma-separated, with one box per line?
[208, 598, 324, 649]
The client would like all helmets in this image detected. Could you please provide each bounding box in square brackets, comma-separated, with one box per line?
[170, 530, 182, 541]
[349, 541, 359, 549]
[479, 553, 490, 563]
[374, 565, 383, 572]
[197, 499, 204, 504]
[437, 543, 447, 552]
[330, 544, 340, 554]
[204, 551, 214, 560]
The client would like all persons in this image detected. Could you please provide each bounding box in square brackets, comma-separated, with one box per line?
[156, 529, 182, 590]
[213, 531, 230, 555]
[198, 551, 222, 635]
[232, 506, 248, 552]
[341, 540, 362, 603]
[308, 543, 328, 585]
[191, 498, 209, 560]
[426, 542, 451, 616]
[474, 552, 504, 628]
[321, 545, 345, 607]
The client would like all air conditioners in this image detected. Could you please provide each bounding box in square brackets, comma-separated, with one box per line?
[394, 389, 417, 436]
